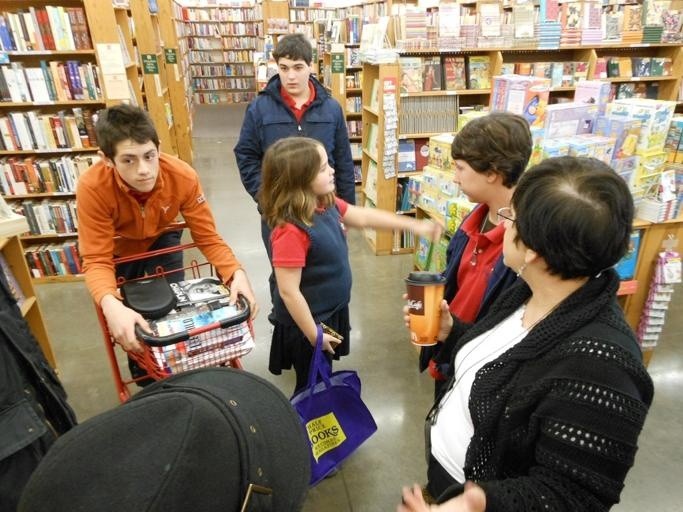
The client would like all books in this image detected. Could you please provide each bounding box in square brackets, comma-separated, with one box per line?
[0, 5, 103, 281]
[173, 2, 279, 107]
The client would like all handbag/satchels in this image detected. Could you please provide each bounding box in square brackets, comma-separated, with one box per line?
[121, 277, 177, 319]
[291, 371, 378, 489]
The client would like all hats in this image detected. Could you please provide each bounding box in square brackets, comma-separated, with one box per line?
[19, 367, 311, 511]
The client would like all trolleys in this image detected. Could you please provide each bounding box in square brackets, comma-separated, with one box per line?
[67, 239, 259, 403]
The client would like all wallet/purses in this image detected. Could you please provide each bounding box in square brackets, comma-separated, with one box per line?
[317, 322, 344, 350]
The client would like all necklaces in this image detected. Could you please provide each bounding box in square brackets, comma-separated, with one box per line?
[424, 405, 440, 426]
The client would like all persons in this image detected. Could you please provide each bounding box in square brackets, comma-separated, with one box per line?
[290, 0, 683, 283]
[419, 111, 533, 402]
[0, 259, 82, 510]
[256, 137, 444, 479]
[391, 154, 655, 512]
[75, 103, 261, 389]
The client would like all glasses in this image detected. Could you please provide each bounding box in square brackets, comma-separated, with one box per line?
[498, 208, 516, 227]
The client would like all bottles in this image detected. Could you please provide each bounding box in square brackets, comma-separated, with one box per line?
[404, 270, 448, 346]
